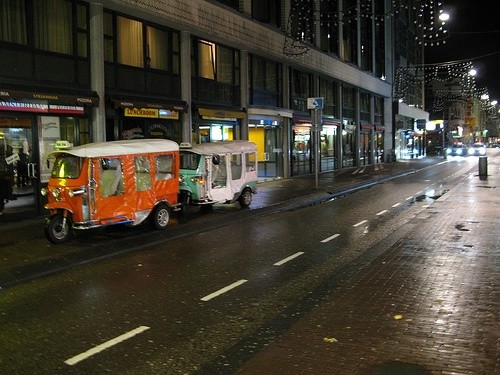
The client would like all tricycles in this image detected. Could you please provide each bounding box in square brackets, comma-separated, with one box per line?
[43, 138, 180, 244]
[176, 139, 259, 207]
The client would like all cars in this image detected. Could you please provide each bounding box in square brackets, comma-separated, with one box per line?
[471, 143, 486, 156]
[450, 143, 466, 156]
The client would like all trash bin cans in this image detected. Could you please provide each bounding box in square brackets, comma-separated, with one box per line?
[479, 157, 487, 176]
